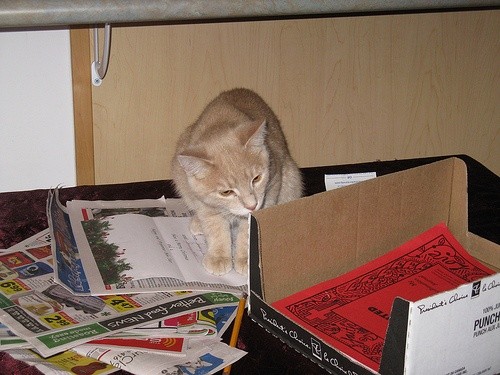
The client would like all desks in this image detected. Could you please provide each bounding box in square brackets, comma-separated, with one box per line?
[0, 154, 500, 375]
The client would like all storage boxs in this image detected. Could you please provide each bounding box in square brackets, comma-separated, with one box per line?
[247, 157, 500, 374]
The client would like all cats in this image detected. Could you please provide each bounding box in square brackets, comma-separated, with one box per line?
[169, 86, 307, 276]
